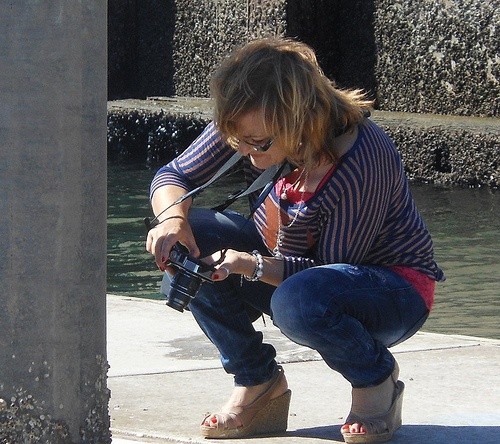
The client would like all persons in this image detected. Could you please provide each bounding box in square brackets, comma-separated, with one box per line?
[142, 37, 449, 444]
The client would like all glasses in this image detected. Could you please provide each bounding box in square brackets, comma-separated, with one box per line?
[230, 127, 284, 152]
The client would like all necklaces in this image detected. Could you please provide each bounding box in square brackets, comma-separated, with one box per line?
[271, 159, 311, 256]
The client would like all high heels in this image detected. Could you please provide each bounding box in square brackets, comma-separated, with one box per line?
[341, 371, 405, 444]
[201, 365, 292, 437]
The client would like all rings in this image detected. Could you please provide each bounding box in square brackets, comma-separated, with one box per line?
[220, 264, 231, 280]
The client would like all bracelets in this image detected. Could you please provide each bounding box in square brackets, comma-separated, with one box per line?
[239, 249, 265, 286]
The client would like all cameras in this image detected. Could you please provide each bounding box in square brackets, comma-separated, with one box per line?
[165, 247, 217, 313]
